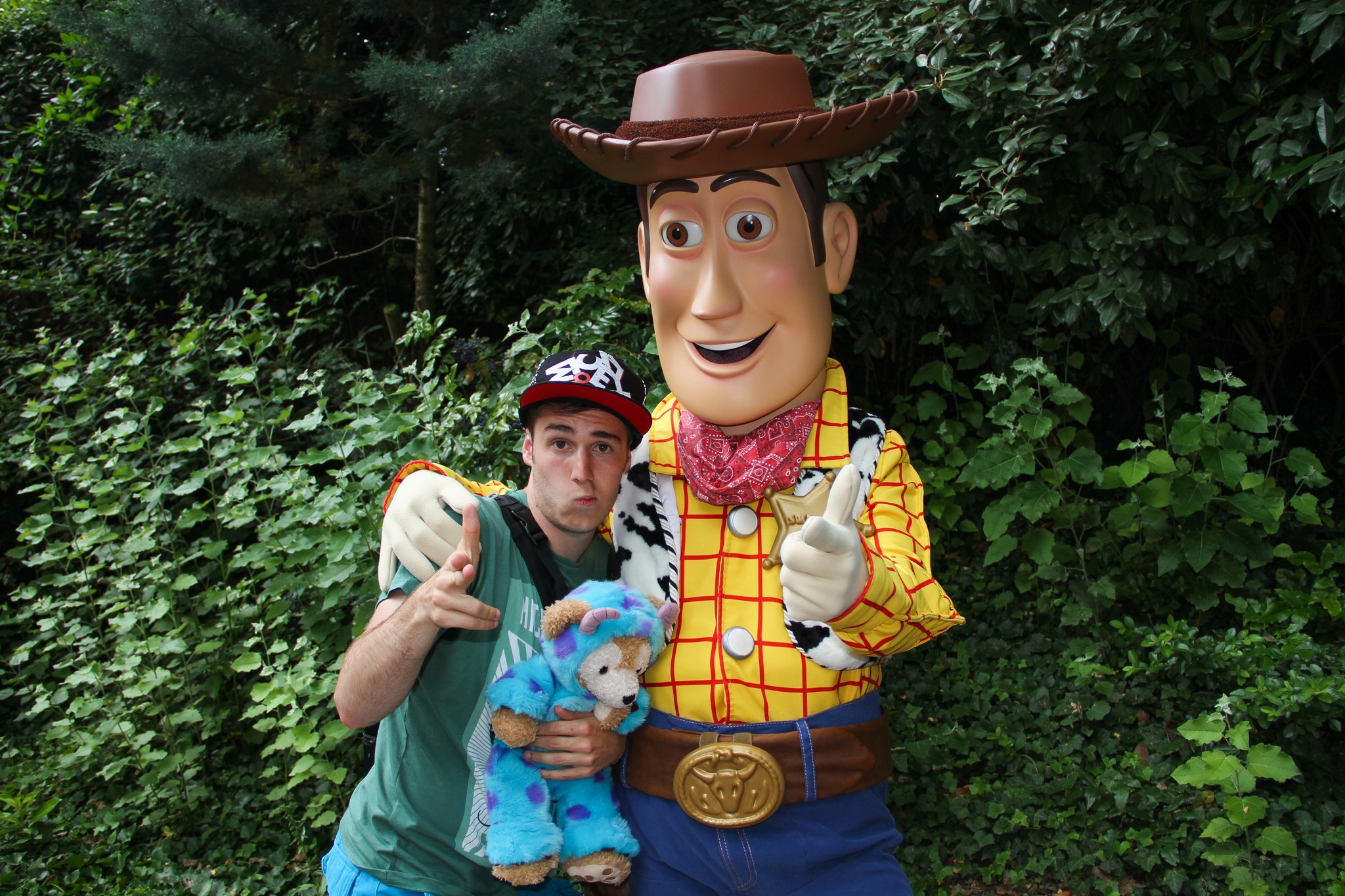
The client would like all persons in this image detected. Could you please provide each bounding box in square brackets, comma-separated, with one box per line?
[321, 348, 653, 896]
[377, 47, 967, 896]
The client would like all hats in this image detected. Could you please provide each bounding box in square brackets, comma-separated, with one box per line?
[520, 350, 651, 450]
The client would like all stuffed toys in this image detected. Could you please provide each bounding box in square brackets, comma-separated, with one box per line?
[482, 578, 680, 885]
